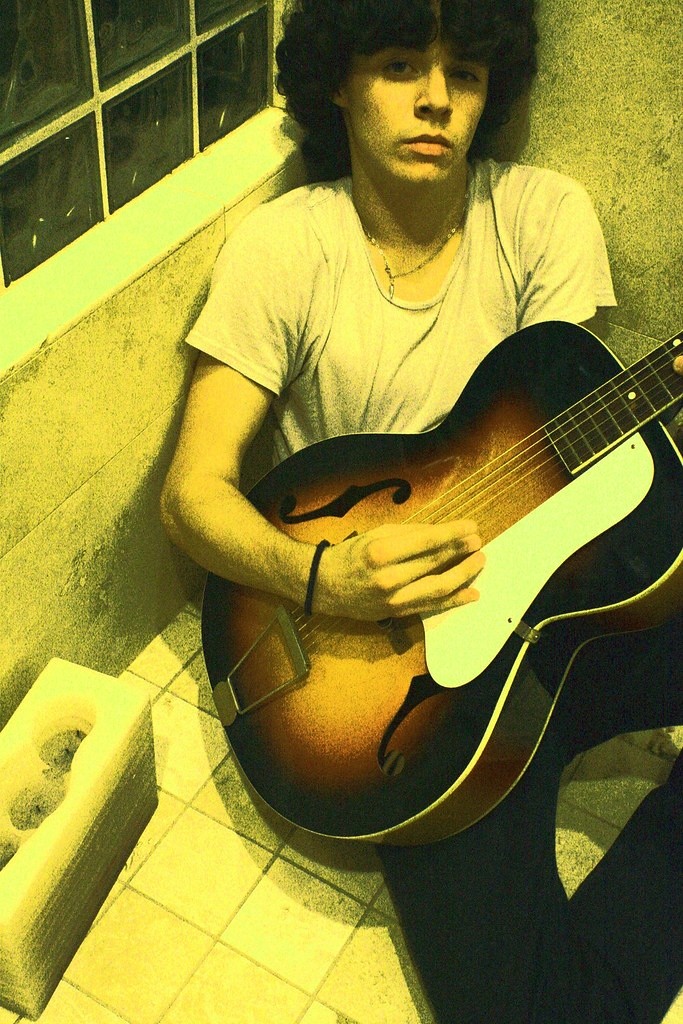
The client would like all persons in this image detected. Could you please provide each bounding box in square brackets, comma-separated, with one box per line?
[162, 1, 683, 1024]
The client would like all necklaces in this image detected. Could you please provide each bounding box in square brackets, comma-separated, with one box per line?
[362, 208, 467, 300]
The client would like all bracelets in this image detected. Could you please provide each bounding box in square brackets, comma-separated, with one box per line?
[303, 539, 329, 617]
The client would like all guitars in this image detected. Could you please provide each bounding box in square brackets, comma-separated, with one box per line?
[200, 320, 683, 843]
